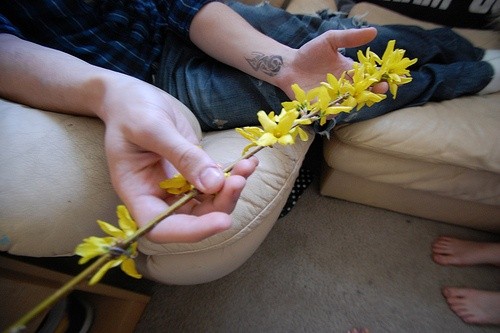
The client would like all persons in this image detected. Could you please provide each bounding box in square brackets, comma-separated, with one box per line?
[0, 0, 500, 245]
[431, 235, 500, 325]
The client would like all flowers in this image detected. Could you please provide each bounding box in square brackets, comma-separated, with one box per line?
[0, 40, 418, 333]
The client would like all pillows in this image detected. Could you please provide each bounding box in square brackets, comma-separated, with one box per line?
[334, 3, 500, 172]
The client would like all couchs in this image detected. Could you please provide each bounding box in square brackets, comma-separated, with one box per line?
[0, 1, 499, 285]
